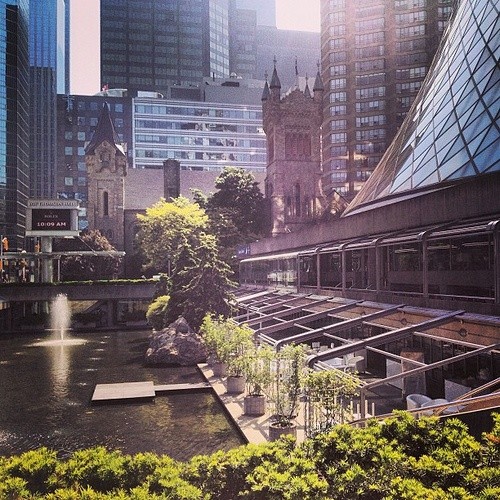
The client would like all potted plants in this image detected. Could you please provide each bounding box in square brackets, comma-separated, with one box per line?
[197, 311, 365, 450]
